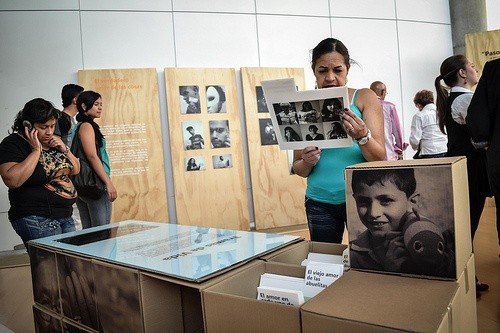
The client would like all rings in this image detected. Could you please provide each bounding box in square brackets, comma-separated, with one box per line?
[347, 126, 355, 133]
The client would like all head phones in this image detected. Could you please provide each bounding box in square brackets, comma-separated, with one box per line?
[23, 120, 32, 129]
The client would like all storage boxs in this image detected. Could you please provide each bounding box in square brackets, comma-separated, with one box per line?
[262, 240, 350, 271]
[28, 219, 310, 333]
[304, 278, 462, 333]
[458, 253, 478, 333]
[344, 155, 472, 280]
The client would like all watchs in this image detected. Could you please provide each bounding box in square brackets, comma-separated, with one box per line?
[353, 128, 371, 145]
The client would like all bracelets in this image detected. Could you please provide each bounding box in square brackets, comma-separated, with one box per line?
[63, 144, 69, 155]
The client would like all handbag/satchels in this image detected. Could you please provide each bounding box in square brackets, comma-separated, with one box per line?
[68, 122, 105, 200]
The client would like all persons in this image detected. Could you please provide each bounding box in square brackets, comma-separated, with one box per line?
[465, 58, 500, 244]
[179, 85, 230, 171]
[277, 98, 348, 142]
[435, 55, 489, 291]
[410, 89, 448, 159]
[53, 83, 85, 231]
[264, 122, 277, 142]
[0, 97, 80, 252]
[370, 81, 409, 161]
[349, 169, 455, 278]
[69, 90, 117, 230]
[292, 37, 385, 244]
